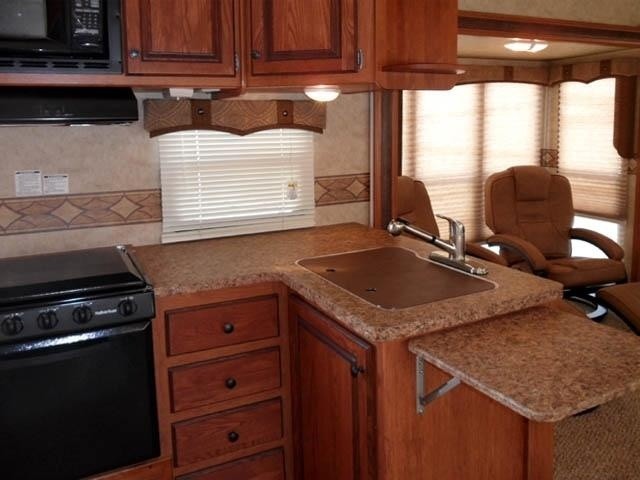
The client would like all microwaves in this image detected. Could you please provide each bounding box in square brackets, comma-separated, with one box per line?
[1, 0, 123, 75]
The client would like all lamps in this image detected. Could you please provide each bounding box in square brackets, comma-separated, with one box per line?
[304, 85, 341, 102]
[504, 40, 548, 52]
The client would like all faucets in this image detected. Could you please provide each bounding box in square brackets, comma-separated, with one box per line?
[387, 213, 489, 274]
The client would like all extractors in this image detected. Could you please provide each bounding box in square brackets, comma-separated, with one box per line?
[0, 86, 140, 128]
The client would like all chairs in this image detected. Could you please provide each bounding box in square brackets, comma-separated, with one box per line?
[397, 176, 509, 266]
[485, 166, 628, 322]
[596, 282, 640, 334]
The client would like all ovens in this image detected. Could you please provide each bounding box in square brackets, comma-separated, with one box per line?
[0, 324, 167, 480]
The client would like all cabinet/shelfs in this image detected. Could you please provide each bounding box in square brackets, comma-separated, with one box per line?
[155, 280, 296, 478]
[249, 0, 377, 91]
[378, 0, 457, 90]
[288, 281, 377, 480]
[125, 1, 245, 93]
[378, 281, 528, 478]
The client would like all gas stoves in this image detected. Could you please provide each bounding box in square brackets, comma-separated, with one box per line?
[0, 242, 157, 346]
